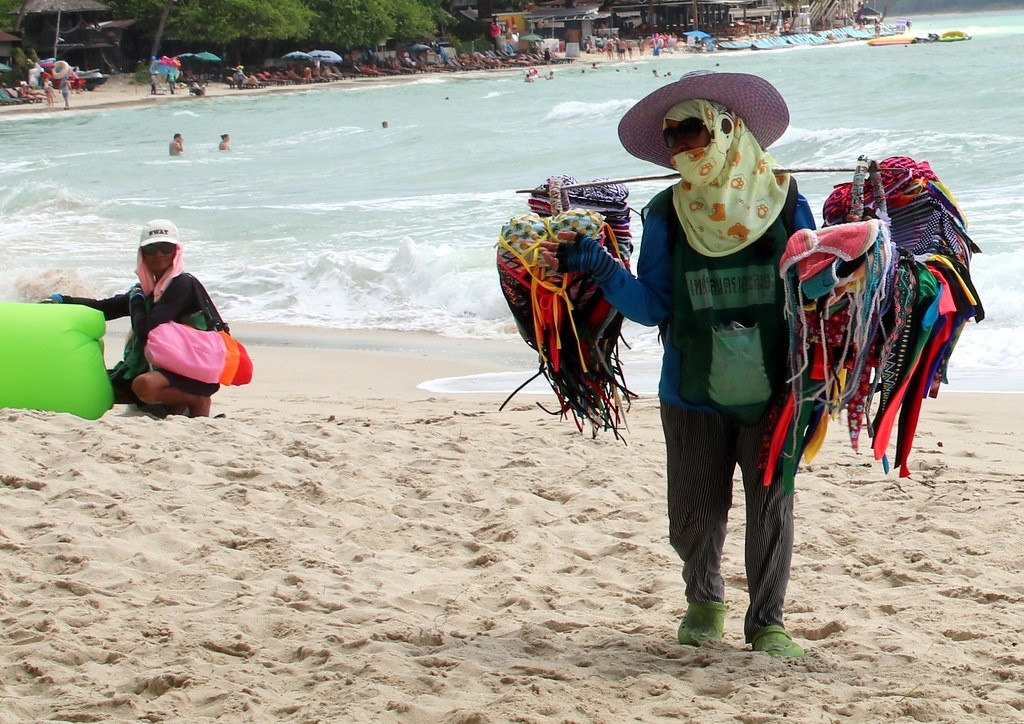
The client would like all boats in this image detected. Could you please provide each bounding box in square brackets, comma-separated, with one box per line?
[39, 69, 109, 91]
[867, 39, 911, 46]
[938, 32, 972, 42]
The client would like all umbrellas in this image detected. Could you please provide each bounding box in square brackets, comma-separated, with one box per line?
[0, 63, 13, 74]
[683, 30, 711, 40]
[149, 51, 222, 74]
[519, 34, 545, 43]
[280, 49, 343, 65]
[404, 43, 431, 52]
[8, 0, 112, 58]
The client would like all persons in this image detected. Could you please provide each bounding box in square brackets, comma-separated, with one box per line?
[231, 55, 345, 87]
[18, 67, 80, 111]
[219, 134, 231, 150]
[351, 39, 556, 84]
[170, 133, 183, 155]
[148, 64, 205, 97]
[694, 36, 714, 54]
[382, 121, 389, 127]
[539, 69, 817, 658]
[581, 62, 720, 78]
[780, 17, 913, 43]
[585, 28, 679, 60]
[38, 218, 231, 418]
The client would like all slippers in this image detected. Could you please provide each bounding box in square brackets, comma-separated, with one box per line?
[678, 600, 729, 646]
[752, 625, 805, 658]
[215, 413, 228, 420]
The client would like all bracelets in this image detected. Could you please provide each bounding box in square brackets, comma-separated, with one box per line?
[50, 294, 64, 303]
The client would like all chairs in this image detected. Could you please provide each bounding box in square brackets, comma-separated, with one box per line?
[177, 50, 576, 89]
[0, 87, 43, 105]
[719, 26, 897, 50]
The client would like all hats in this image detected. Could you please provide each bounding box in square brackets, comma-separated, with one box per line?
[140, 219, 181, 246]
[617, 70, 790, 171]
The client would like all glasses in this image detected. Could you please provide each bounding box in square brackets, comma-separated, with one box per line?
[142, 243, 173, 254]
[663, 116, 704, 149]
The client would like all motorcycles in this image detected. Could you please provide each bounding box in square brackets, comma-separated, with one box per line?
[912, 33, 940, 43]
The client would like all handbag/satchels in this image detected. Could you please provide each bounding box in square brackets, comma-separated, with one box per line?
[144, 273, 253, 386]
